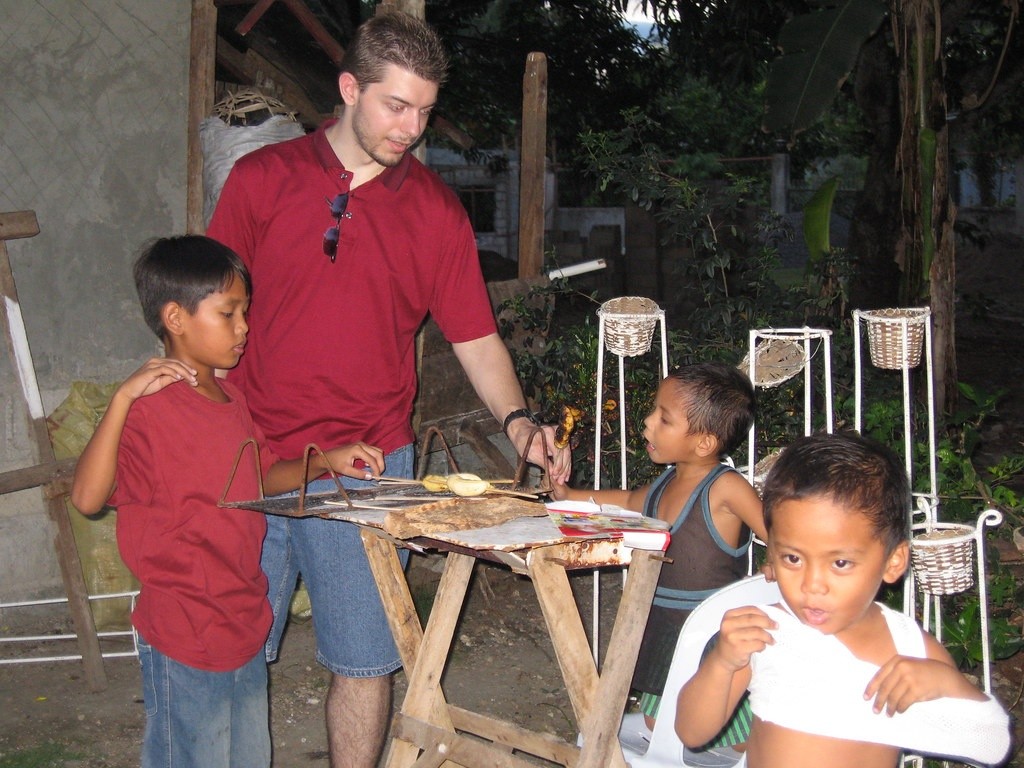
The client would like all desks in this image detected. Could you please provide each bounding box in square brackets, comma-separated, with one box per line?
[327, 498, 672, 767]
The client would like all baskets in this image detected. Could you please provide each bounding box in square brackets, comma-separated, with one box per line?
[860, 308, 929, 370]
[911, 528, 977, 596]
[601, 297, 661, 356]
[738, 339, 806, 390]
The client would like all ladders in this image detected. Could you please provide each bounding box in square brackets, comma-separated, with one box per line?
[1, 207, 110, 697]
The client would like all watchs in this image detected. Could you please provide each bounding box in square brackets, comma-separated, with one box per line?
[503, 409, 540, 437]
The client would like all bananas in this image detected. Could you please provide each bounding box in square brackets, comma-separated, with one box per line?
[443, 472, 490, 497]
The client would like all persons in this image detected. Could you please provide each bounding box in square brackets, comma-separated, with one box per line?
[71, 235, 385, 768]
[207, 13, 573, 768]
[539, 362, 777, 753]
[674, 431, 1011, 768]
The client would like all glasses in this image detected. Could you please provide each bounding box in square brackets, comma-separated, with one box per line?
[323, 192, 349, 263]
